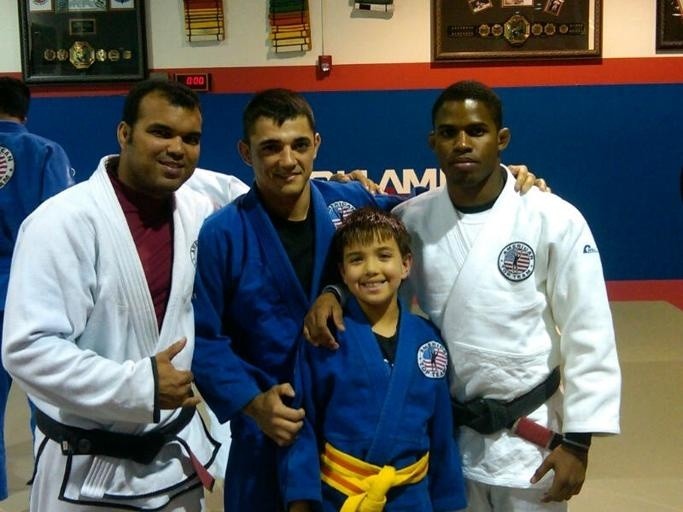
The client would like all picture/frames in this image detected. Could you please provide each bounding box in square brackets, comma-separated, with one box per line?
[655, 0, 683, 53]
[431, 0, 603, 62]
[17, 1, 148, 86]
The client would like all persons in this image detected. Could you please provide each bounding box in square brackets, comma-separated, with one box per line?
[1, 70, 77, 505]
[192, 86, 554, 512]
[0, 76, 387, 512]
[278, 207, 472, 512]
[300, 80, 621, 512]
